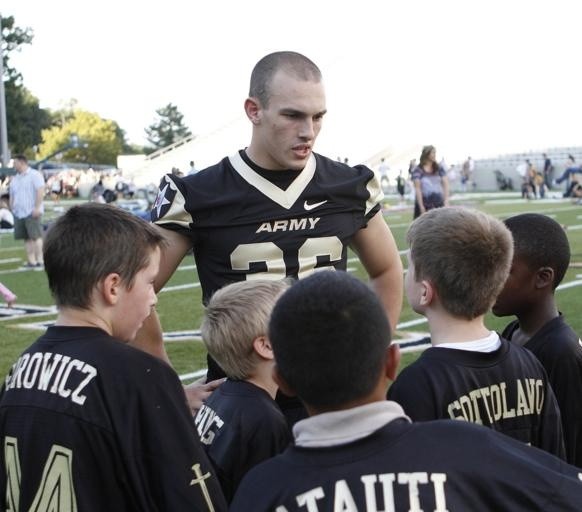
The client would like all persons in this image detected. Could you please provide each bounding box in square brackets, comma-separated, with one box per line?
[191, 277, 293, 506]
[1, 202, 227, 510]
[338, 144, 582, 217]
[232, 270, 582, 511]
[1, 155, 199, 271]
[491, 214, 582, 467]
[121, 52, 406, 436]
[385, 207, 567, 463]
